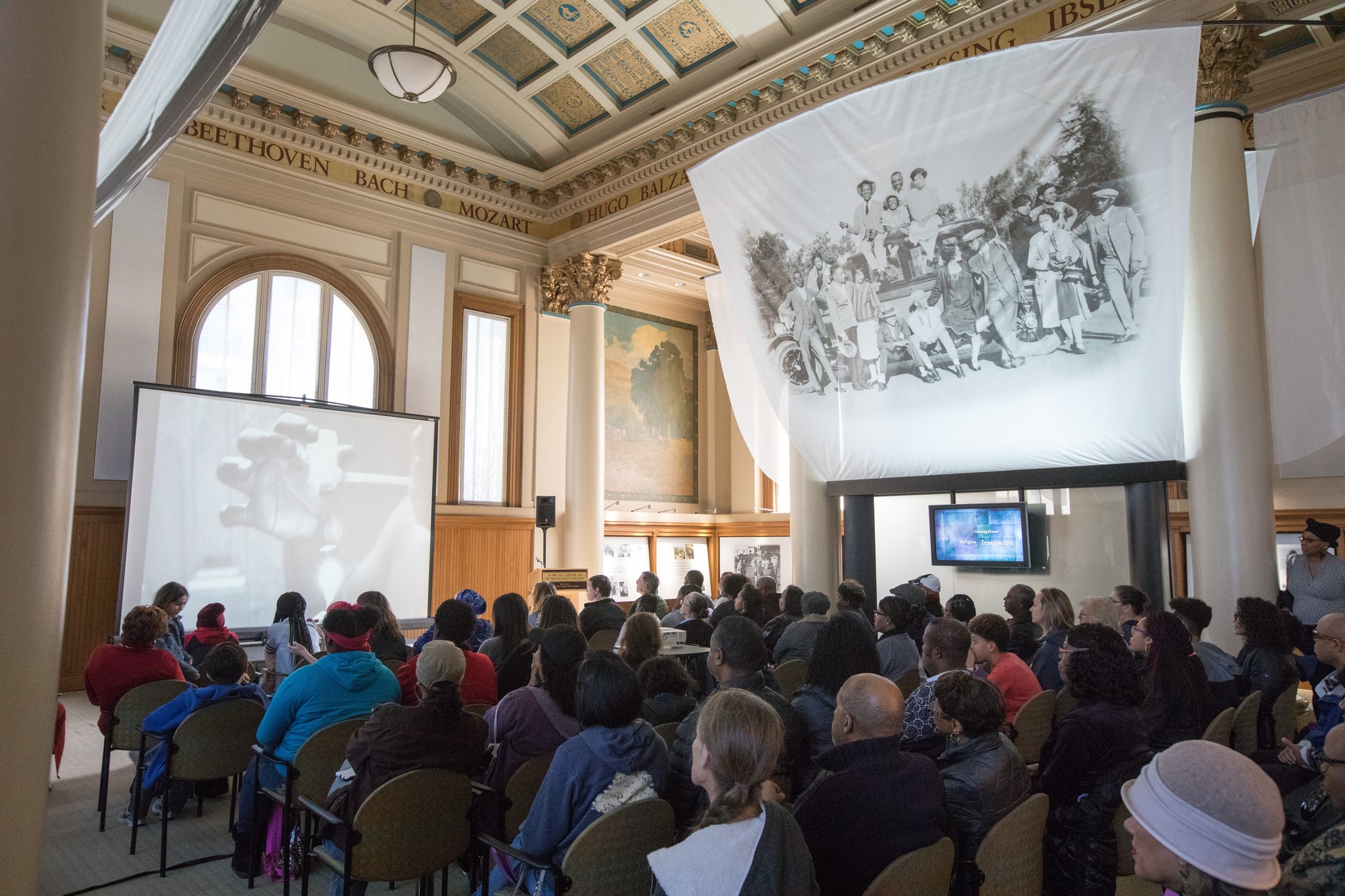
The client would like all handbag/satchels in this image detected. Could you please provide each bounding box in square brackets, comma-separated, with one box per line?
[264, 806, 295, 883]
[754, 572, 757, 576]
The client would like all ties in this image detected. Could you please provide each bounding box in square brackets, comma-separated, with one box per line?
[866, 201, 869, 215]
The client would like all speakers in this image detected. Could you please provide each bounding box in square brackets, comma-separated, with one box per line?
[536, 496, 556, 528]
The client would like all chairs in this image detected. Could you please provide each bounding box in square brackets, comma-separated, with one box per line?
[1200, 681, 1298, 758]
[96, 629, 1058, 896]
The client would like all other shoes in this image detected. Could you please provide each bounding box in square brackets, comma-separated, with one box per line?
[231, 856, 260, 878]
[816, 389, 825, 396]
[1000, 356, 1026, 369]
[1070, 342, 1087, 354]
[1114, 333, 1140, 343]
[153, 797, 176, 820]
[970, 360, 981, 371]
[852, 364, 965, 391]
[872, 254, 938, 280]
[834, 386, 846, 392]
[119, 807, 147, 827]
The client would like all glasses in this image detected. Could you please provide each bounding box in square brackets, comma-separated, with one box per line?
[1058, 647, 1074, 655]
[942, 608, 951, 613]
[1079, 612, 1088, 620]
[1312, 629, 1345, 646]
[679, 600, 692, 608]
[1298, 536, 1323, 543]
[636, 579, 643, 582]
[1131, 626, 1147, 636]
[1234, 613, 1240, 621]
[1312, 750, 1345, 772]
[892, 595, 895, 597]
[927, 700, 953, 721]
[873, 610, 886, 617]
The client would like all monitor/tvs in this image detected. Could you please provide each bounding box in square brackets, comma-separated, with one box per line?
[927, 502, 1032, 570]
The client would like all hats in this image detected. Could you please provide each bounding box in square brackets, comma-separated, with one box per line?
[416, 640, 466, 690]
[456, 588, 487, 615]
[1120, 740, 1284, 890]
[962, 228, 986, 242]
[801, 591, 830, 611]
[529, 623, 588, 660]
[908, 574, 941, 592]
[1092, 189, 1119, 200]
[196, 603, 225, 629]
[686, 545, 693, 550]
[857, 179, 876, 196]
[1305, 518, 1341, 543]
[890, 583, 924, 605]
[839, 340, 858, 358]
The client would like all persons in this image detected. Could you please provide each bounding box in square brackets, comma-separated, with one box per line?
[778, 169, 1149, 398]
[1284, 518, 1345, 637]
[609, 544, 777, 598]
[84, 572, 1345, 896]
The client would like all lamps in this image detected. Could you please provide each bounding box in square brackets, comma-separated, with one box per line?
[367, 0, 458, 104]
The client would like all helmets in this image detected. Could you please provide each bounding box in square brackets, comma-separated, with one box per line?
[772, 563, 778, 566]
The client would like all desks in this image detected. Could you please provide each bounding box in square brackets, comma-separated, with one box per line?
[657, 645, 710, 678]
